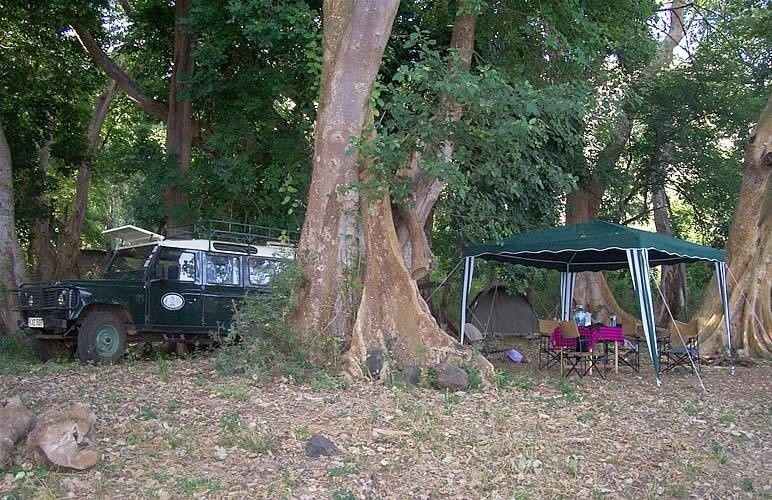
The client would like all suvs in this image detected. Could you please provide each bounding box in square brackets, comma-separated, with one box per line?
[572, 305, 585, 325]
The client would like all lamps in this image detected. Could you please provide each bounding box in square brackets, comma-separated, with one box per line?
[538, 319, 700, 379]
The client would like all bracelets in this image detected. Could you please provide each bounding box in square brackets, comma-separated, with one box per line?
[506, 349, 523, 363]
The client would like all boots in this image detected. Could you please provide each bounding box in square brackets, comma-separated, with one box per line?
[13, 221, 303, 367]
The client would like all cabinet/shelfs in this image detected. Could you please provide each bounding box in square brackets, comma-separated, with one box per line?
[571, 305, 593, 326]
[609, 313, 617, 326]
